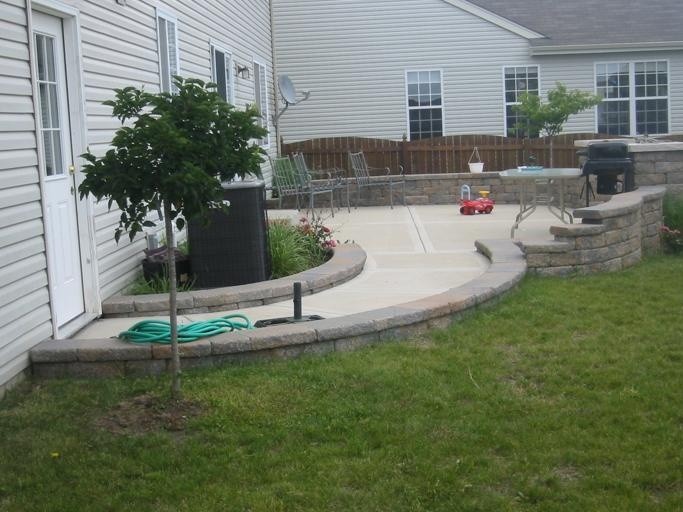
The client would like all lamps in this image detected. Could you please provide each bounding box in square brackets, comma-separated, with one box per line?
[236, 65, 250, 80]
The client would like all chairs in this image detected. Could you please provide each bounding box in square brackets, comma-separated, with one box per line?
[270, 147, 407, 221]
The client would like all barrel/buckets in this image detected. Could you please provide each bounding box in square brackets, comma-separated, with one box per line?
[467, 146, 485, 173]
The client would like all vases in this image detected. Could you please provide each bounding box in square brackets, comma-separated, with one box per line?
[468, 163, 485, 173]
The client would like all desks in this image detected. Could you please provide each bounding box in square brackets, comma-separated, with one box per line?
[499, 168, 584, 238]
